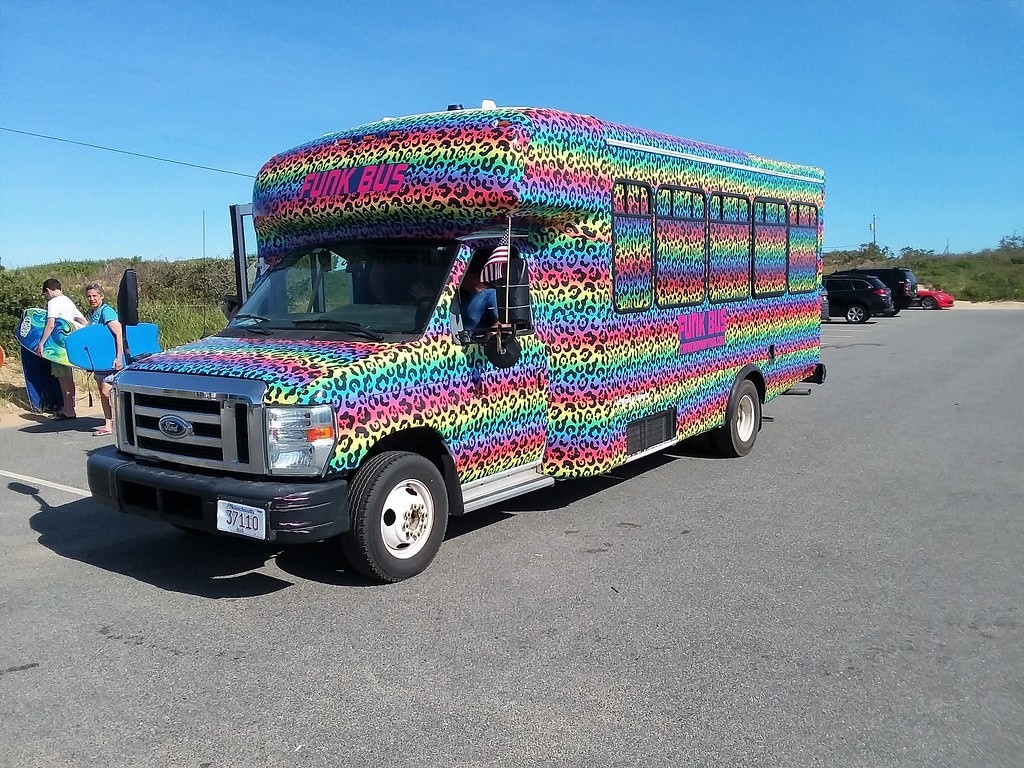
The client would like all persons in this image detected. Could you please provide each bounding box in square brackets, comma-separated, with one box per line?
[412, 253, 512, 338]
[85, 285, 123, 435]
[34, 279, 87, 419]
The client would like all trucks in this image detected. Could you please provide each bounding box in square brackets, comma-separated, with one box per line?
[86, 103, 826, 582]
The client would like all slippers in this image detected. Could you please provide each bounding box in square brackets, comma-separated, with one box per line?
[48, 413, 77, 421]
[93, 429, 112, 436]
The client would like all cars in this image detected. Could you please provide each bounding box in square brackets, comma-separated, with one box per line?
[831, 266, 918, 316]
[906, 284, 955, 310]
[822, 275, 894, 323]
[821, 286, 829, 321]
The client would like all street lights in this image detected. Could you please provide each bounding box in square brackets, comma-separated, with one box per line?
[870, 214, 876, 244]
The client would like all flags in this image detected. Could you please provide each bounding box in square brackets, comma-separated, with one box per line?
[480, 227, 509, 282]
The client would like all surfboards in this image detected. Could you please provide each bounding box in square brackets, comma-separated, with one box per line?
[13, 305, 164, 413]
